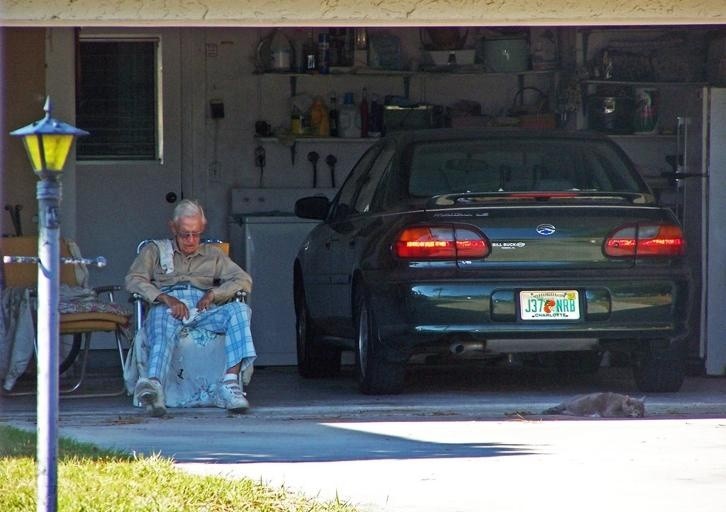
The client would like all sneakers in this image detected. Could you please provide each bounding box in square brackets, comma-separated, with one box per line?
[214, 385, 249, 410]
[135, 377, 167, 417]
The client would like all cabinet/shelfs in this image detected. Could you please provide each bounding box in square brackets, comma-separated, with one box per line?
[250, 67, 710, 146]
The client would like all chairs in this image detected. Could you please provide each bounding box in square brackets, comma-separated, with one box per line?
[407, 155, 601, 199]
[1, 229, 132, 399]
[126, 236, 249, 400]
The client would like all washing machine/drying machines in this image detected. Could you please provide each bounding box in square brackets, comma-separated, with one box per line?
[225, 185, 338, 365]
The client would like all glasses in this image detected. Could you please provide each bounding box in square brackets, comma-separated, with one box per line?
[179, 231, 203, 237]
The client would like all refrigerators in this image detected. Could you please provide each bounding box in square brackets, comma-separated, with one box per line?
[671, 84, 725, 377]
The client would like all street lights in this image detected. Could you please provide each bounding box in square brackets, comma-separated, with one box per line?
[9, 97, 90, 512]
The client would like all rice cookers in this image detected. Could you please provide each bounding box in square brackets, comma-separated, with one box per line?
[586, 88, 633, 135]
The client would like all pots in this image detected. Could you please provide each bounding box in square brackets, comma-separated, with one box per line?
[478, 31, 530, 72]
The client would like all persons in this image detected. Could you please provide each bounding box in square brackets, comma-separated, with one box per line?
[122, 198, 256, 417]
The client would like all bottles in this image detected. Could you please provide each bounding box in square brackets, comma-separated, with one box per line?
[304, 31, 338, 74]
[329, 88, 380, 138]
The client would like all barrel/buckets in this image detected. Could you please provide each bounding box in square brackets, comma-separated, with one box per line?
[310, 95, 329, 138]
[337, 92, 365, 140]
[268, 28, 291, 71]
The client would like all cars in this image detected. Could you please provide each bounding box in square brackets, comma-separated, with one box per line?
[291, 125, 695, 393]
